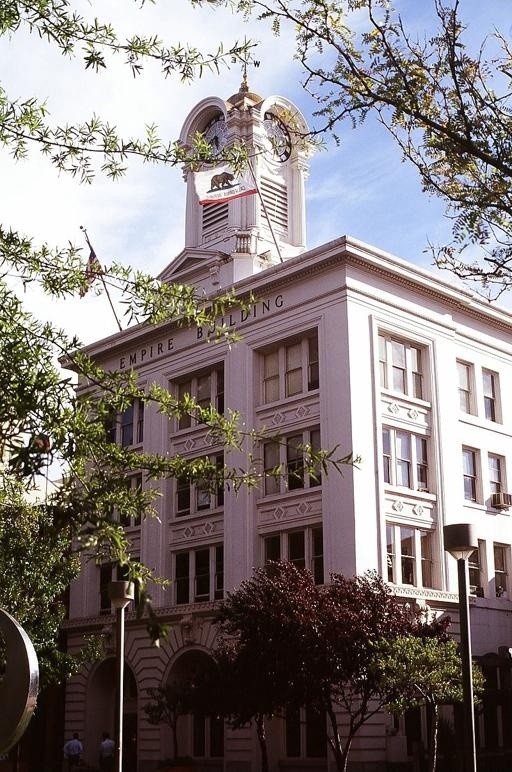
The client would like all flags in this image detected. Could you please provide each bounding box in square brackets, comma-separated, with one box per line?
[194, 166, 256, 204]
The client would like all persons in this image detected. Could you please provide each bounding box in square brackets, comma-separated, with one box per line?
[64, 732, 83, 765]
[98, 732, 115, 767]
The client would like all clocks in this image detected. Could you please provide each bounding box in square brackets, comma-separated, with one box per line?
[257, 113, 291, 164]
[198, 114, 227, 165]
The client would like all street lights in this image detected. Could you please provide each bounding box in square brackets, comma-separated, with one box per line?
[443, 523, 479, 772]
[105, 581, 135, 772]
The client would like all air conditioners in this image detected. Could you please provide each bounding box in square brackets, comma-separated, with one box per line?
[491, 493, 512, 510]
